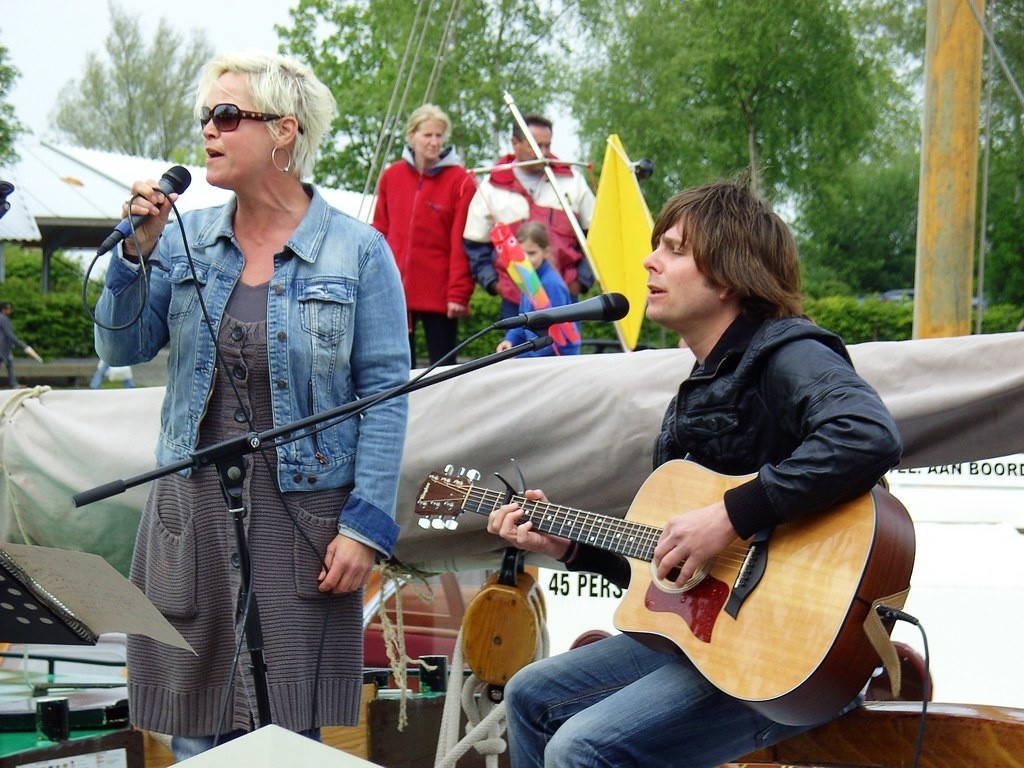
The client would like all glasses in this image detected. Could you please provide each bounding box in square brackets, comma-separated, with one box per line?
[199, 104, 305, 136]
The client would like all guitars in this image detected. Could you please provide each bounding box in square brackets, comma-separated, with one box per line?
[412, 454, 917, 731]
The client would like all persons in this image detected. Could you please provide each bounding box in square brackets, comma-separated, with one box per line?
[93, 43, 411, 768]
[497, 221, 581, 357]
[462, 116, 598, 332]
[488, 167, 902, 768]
[373, 104, 478, 368]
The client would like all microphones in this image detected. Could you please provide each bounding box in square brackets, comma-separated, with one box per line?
[492, 292, 630, 330]
[96, 166, 191, 255]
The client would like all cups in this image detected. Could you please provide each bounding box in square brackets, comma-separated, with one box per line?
[418, 654, 447, 693]
[37, 697, 71, 742]
